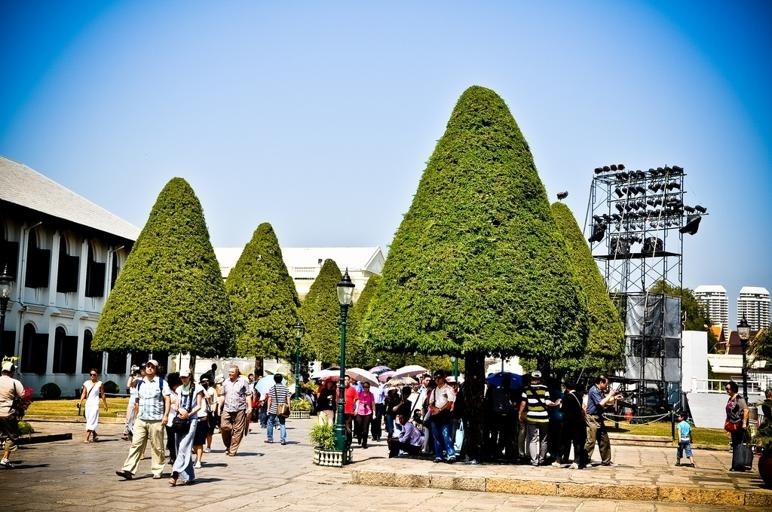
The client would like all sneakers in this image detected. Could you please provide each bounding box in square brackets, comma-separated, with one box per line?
[275, 425, 281, 430]
[281, 439, 287, 445]
[225, 447, 230, 455]
[182, 479, 193, 485]
[674, 462, 681, 466]
[152, 469, 164, 479]
[192, 456, 202, 469]
[686, 463, 695, 467]
[115, 469, 133, 480]
[263, 440, 274, 444]
[204, 446, 212, 453]
[168, 477, 177, 487]
[357, 434, 614, 470]
[229, 452, 236, 457]
[0, 457, 15, 469]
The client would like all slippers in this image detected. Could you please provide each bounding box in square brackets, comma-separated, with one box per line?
[84, 438, 92, 444]
[93, 434, 99, 442]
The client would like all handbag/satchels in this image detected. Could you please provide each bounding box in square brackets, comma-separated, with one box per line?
[171, 414, 192, 437]
[429, 400, 451, 426]
[276, 402, 291, 419]
[455, 418, 466, 452]
[724, 417, 742, 433]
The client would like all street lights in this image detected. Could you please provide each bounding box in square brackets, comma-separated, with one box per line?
[0, 265, 14, 348]
[333, 266, 355, 451]
[450, 356, 459, 386]
[294, 321, 304, 400]
[738, 311, 753, 407]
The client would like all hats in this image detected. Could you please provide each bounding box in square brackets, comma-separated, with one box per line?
[145, 359, 159, 367]
[530, 370, 542, 378]
[178, 367, 192, 378]
[213, 375, 226, 384]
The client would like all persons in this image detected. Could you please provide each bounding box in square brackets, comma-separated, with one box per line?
[299, 375, 398, 449]
[762, 388, 771, 423]
[115, 358, 292, 486]
[384, 368, 623, 471]
[674, 411, 696, 466]
[1, 361, 27, 469]
[724, 381, 753, 472]
[76, 368, 110, 443]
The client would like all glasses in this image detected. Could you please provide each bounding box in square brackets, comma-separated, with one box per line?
[89, 373, 96, 376]
